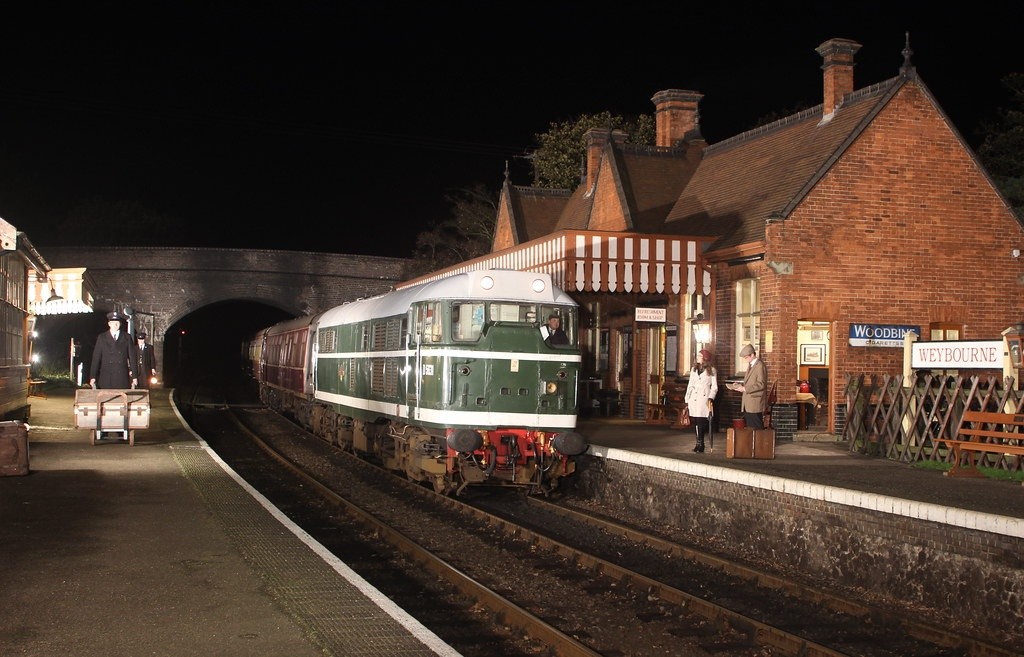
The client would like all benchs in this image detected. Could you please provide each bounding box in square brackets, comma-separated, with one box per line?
[934, 411, 1024, 477]
[642, 383, 691, 429]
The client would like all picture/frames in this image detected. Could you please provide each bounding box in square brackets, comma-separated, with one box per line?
[800, 344, 826, 366]
[811, 330, 823, 340]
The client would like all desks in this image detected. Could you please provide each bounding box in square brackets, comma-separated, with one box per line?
[796, 392, 817, 432]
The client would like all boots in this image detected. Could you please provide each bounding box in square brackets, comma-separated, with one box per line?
[692, 425, 699, 452]
[697, 425, 705, 452]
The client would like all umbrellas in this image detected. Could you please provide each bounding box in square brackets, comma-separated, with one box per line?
[708, 400, 715, 452]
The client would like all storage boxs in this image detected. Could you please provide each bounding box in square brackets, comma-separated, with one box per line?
[73, 388, 150, 431]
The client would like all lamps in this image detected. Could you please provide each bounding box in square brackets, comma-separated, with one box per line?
[1005, 323, 1023, 368]
[45, 277, 63, 301]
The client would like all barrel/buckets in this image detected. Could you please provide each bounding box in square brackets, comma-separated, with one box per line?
[732, 418, 745, 429]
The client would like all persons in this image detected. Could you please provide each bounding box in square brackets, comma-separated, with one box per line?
[88, 312, 139, 441]
[129, 330, 157, 409]
[729, 344, 768, 429]
[542, 313, 570, 345]
[683, 350, 717, 452]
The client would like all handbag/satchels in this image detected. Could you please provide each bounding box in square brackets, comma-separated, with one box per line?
[680, 407, 690, 425]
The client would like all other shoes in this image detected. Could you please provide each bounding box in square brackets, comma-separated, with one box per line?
[118, 433, 124, 441]
[101, 433, 108, 439]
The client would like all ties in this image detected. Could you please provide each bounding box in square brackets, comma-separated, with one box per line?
[113, 334, 117, 341]
[140, 345, 142, 350]
[550, 330, 553, 336]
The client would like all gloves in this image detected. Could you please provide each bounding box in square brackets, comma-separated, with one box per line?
[709, 398, 713, 402]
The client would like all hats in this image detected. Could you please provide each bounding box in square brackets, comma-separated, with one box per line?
[549, 314, 560, 320]
[700, 350, 711, 361]
[106, 312, 123, 321]
[136, 332, 146, 339]
[740, 344, 754, 357]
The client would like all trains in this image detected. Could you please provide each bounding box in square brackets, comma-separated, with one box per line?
[238, 270, 585, 497]
